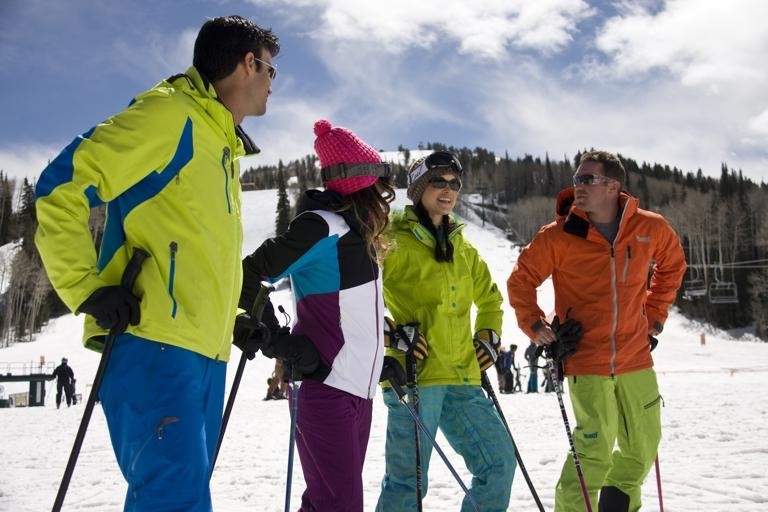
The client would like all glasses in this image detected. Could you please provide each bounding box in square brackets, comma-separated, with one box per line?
[572, 173, 615, 185]
[428, 177, 460, 192]
[254, 57, 278, 79]
[405, 152, 462, 184]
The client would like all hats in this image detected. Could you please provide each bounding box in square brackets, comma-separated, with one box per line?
[313, 119, 382, 194]
[406, 155, 462, 205]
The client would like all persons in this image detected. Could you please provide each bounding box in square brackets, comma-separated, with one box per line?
[372, 150, 519, 512]
[503, 344, 517, 394]
[52, 357, 74, 409]
[506, 149, 689, 512]
[239, 120, 394, 512]
[494, 347, 505, 393]
[514, 368, 526, 392]
[32, 13, 284, 512]
[524, 339, 539, 393]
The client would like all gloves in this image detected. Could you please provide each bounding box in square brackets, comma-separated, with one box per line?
[232, 314, 271, 361]
[474, 328, 501, 372]
[274, 333, 320, 380]
[379, 356, 408, 387]
[78, 286, 142, 334]
[546, 316, 584, 362]
[383, 316, 429, 360]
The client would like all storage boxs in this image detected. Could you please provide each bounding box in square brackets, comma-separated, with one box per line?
[9, 392, 27, 408]
